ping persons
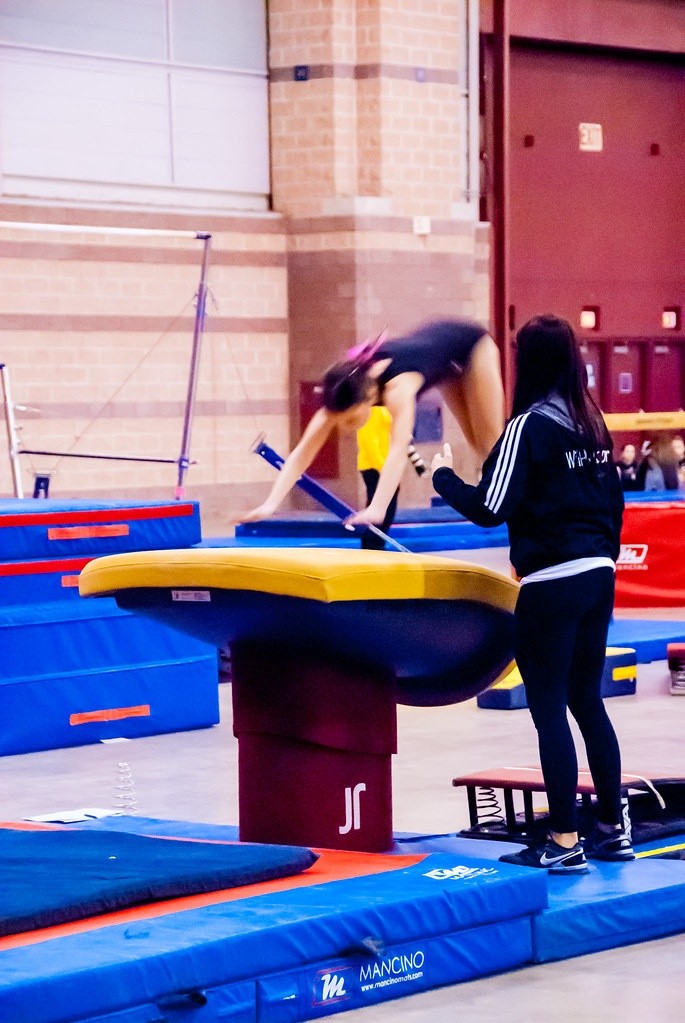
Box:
[238,317,504,524]
[615,443,639,491]
[430,312,632,873]
[636,431,685,490]
[357,404,427,551]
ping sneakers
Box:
[499,834,589,875]
[580,825,635,861]
